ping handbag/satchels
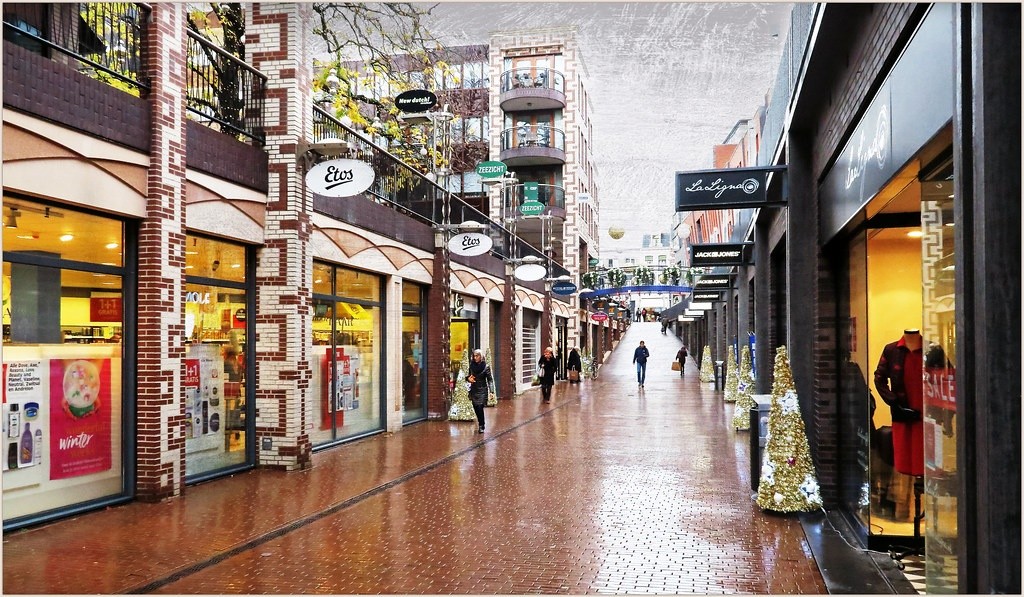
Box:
[569,367,578,380]
[672,362,681,371]
[661,328,665,332]
[539,367,544,377]
[678,350,681,358]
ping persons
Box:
[873,328,956,473]
[567,345,582,383]
[634,306,661,322]
[633,341,650,388]
[537,346,558,404]
[661,314,669,335]
[465,348,495,432]
[675,346,688,376]
[224,348,245,446]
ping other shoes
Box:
[638,384,642,386]
[546,401,550,404]
[642,385,644,387]
[543,400,546,403]
[479,426,484,433]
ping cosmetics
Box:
[7,401,43,470]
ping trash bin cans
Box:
[714,361,724,394]
[748,394,772,501]
